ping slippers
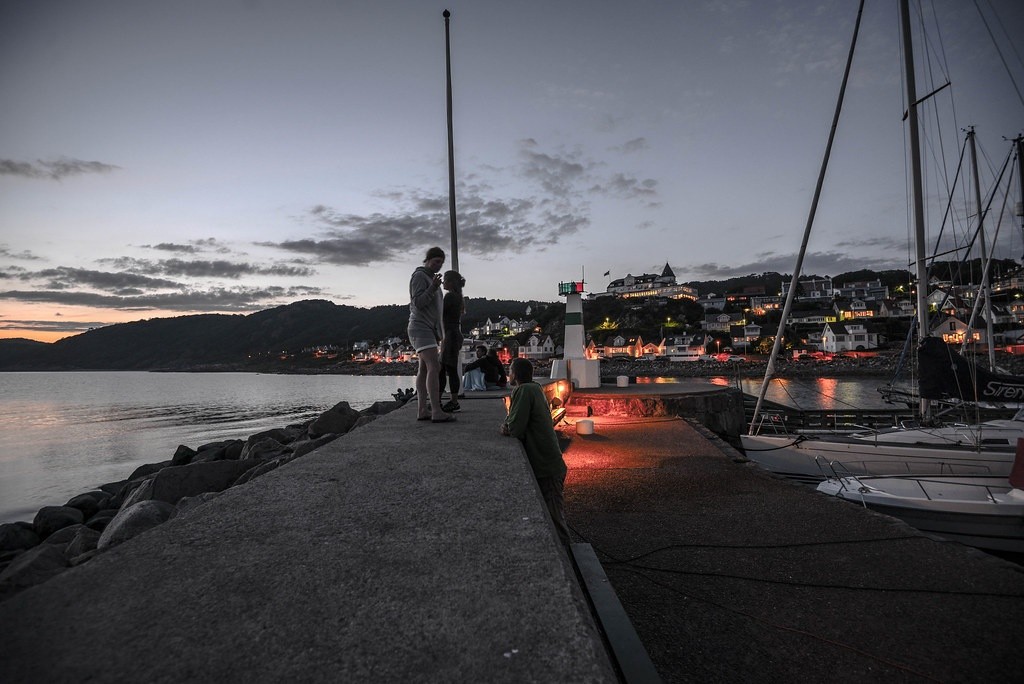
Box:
[417,413,432,420]
[431,411,457,423]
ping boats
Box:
[814,456,1024,517]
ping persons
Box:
[500,358,574,544]
[391,388,414,404]
[462,345,507,392]
[407,247,457,423]
[427,270,466,413]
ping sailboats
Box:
[739,0,1024,485]
[875,125,1024,416]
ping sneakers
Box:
[442,399,461,413]
[428,399,442,411]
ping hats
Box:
[423,247,444,263]
